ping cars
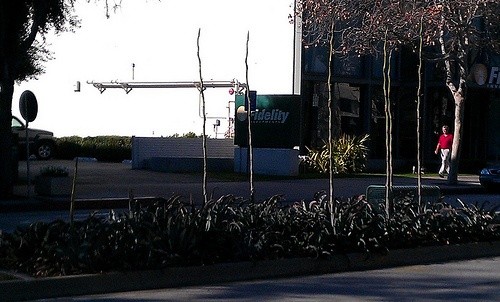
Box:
[479,160,500,190]
[10,126,58,159]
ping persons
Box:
[434,125,454,177]
